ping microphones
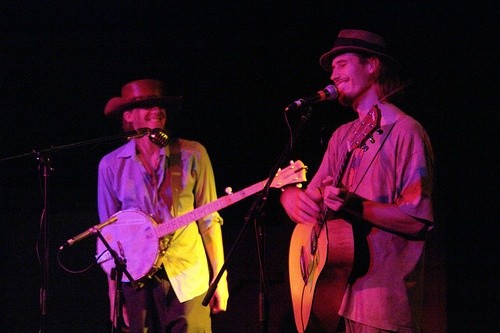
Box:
[139,128,170,147]
[285,85,338,110]
[57,217,117,252]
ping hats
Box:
[105,79,181,117]
[319,30,401,75]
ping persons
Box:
[281,30,435,333]
[98,81,230,333]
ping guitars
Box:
[96,160,308,286]
[288,104,384,333]
